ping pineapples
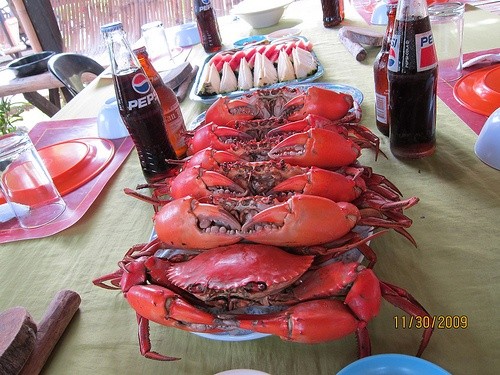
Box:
[198,47,317,96]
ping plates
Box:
[232,27,302,48]
[189,35,324,104]
[187,82,364,132]
[149,223,375,343]
[336,353,453,375]
[452,63,500,117]
[0,136,116,210]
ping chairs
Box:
[47,53,103,98]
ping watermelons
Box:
[209,39,313,74]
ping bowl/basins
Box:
[8,50,56,78]
[175,22,200,47]
[473,106,500,170]
[370,3,391,25]
[229,0,287,29]
[97,96,130,140]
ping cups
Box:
[140,21,176,76]
[428,2,466,84]
[0,131,67,229]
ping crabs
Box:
[91,85,437,357]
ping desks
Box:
[0,0,500,375]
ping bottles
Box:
[100,21,179,185]
[321,0,345,28]
[193,0,223,54]
[373,0,399,137]
[386,0,438,158]
[131,44,192,158]
